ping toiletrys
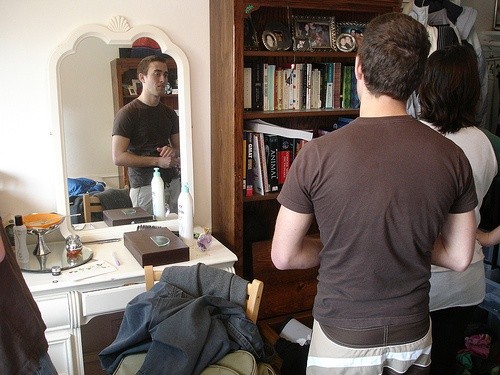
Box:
[3,217,14,252]
[13,215,30,263]
[151,168,164,220]
[178,183,194,246]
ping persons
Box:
[271,11,480,375]
[417,47,500,375]
[0,215,58,375]
[300,24,322,47]
[112,56,180,214]
[339,28,363,50]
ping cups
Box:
[166,203,170,216]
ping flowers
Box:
[194,228,213,252]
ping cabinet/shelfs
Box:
[110,58,178,188]
[209,0,403,375]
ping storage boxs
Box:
[123,227,190,268]
[102,207,154,227]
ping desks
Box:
[19,224,238,375]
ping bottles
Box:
[177,184,194,247]
[13,215,30,264]
[151,168,165,220]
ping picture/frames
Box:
[243,5,371,52]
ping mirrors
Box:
[51,15,196,237]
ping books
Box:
[242,117,355,196]
[244,62,360,113]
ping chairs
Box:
[83,185,130,222]
[144,265,264,326]
